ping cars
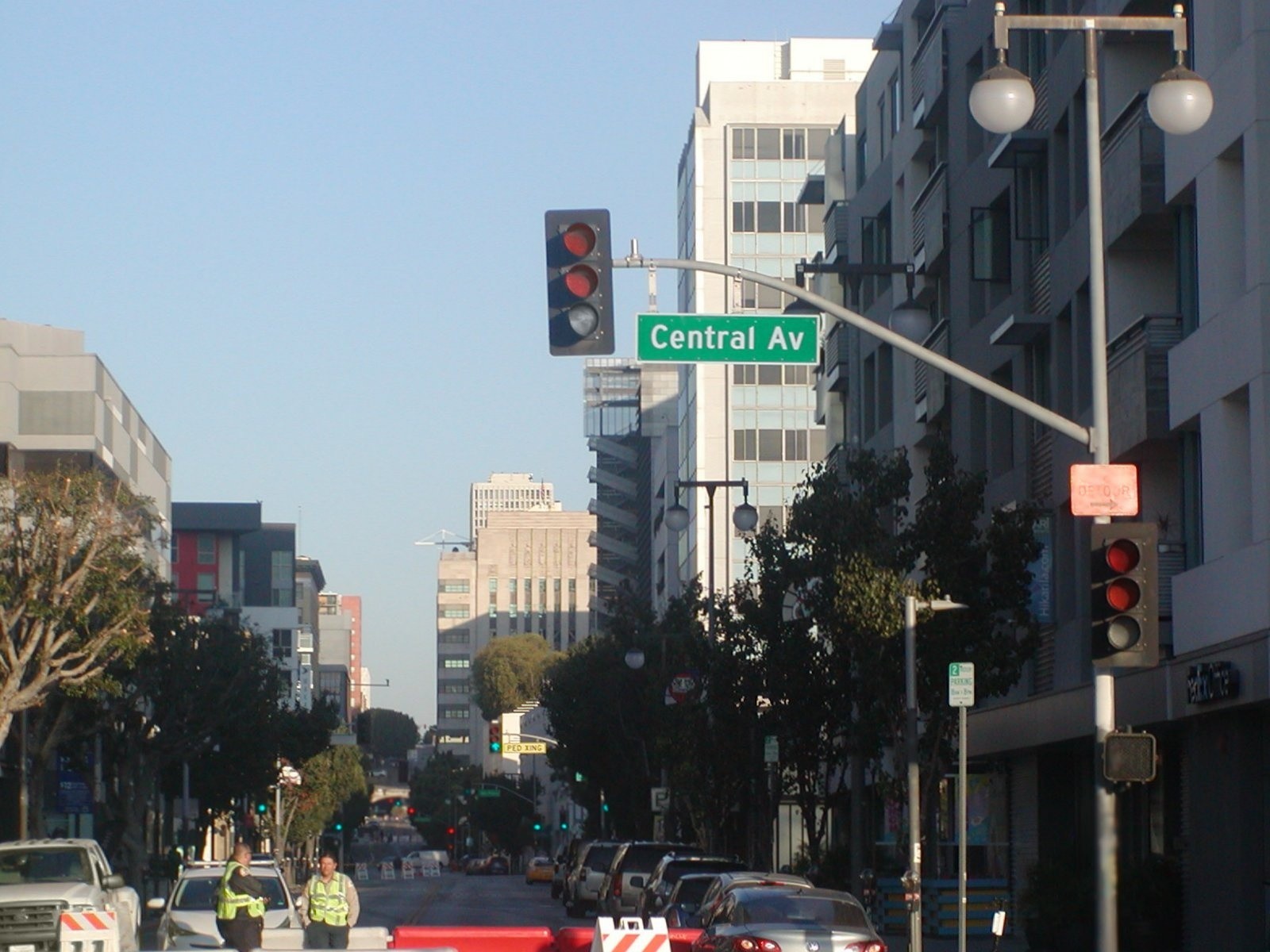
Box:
[527,857,556,885]
[158,859,306,950]
[379,851,512,876]
[698,872,838,928]
[691,886,887,952]
[654,873,720,934]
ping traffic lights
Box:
[389,797,402,807]
[333,821,343,833]
[406,807,418,815]
[543,208,618,357]
[489,723,502,755]
[1102,732,1158,785]
[532,814,543,832]
[255,796,269,816]
[447,826,455,836]
[559,809,569,831]
[463,780,472,797]
[1091,518,1160,671]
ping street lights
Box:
[663,475,760,844]
[906,593,965,952]
[966,1,1215,952]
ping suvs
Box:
[565,841,626,917]
[594,841,708,920]
[630,852,751,928]
[549,842,574,898]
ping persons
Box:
[301,851,360,950]
[551,855,568,900]
[168,843,192,888]
[215,842,271,952]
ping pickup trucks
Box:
[0,836,142,952]
